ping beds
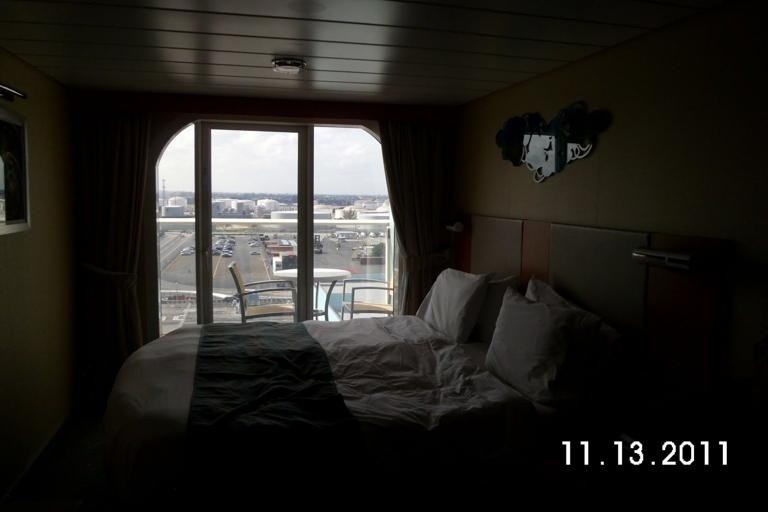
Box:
[102,315,642,508]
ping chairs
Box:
[228,260,395,325]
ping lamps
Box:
[445,222,465,234]
[270,57,307,75]
[632,247,694,284]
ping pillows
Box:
[413,265,623,411]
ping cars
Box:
[180,245,195,256]
[248,232,270,247]
[273,233,277,239]
[250,251,261,255]
[212,235,235,258]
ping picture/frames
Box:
[0,104,31,237]
